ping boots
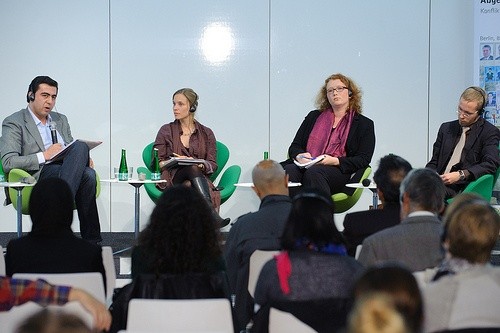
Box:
[191,177,231,230]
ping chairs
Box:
[137,140,240,205]
[331,164,373,212]
[0,155,101,215]
[446,168,500,204]
[0,247,327,333]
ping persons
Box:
[0,154,500,333]
[282,73,375,210]
[1,76,103,251]
[152,88,231,229]
[424,85,500,201]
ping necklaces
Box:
[181,127,195,136]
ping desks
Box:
[100,179,167,255]
[346,183,378,210]
[0,180,35,237]
[233,182,301,188]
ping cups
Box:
[127,166,133,179]
[114,168,119,179]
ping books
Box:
[159,158,205,170]
[50,138,103,162]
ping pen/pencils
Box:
[296,156,316,160]
[173,153,188,158]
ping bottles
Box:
[264,152,268,160]
[0,152,6,182]
[150,150,161,181]
[119,149,128,181]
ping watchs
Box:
[458,170,465,180]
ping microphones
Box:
[362,179,371,187]
[49,121,57,144]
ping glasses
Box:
[327,86,349,94]
[456,106,478,118]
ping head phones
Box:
[27,84,35,100]
[347,80,353,97]
[470,87,485,115]
[189,94,197,112]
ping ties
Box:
[444,126,471,173]
